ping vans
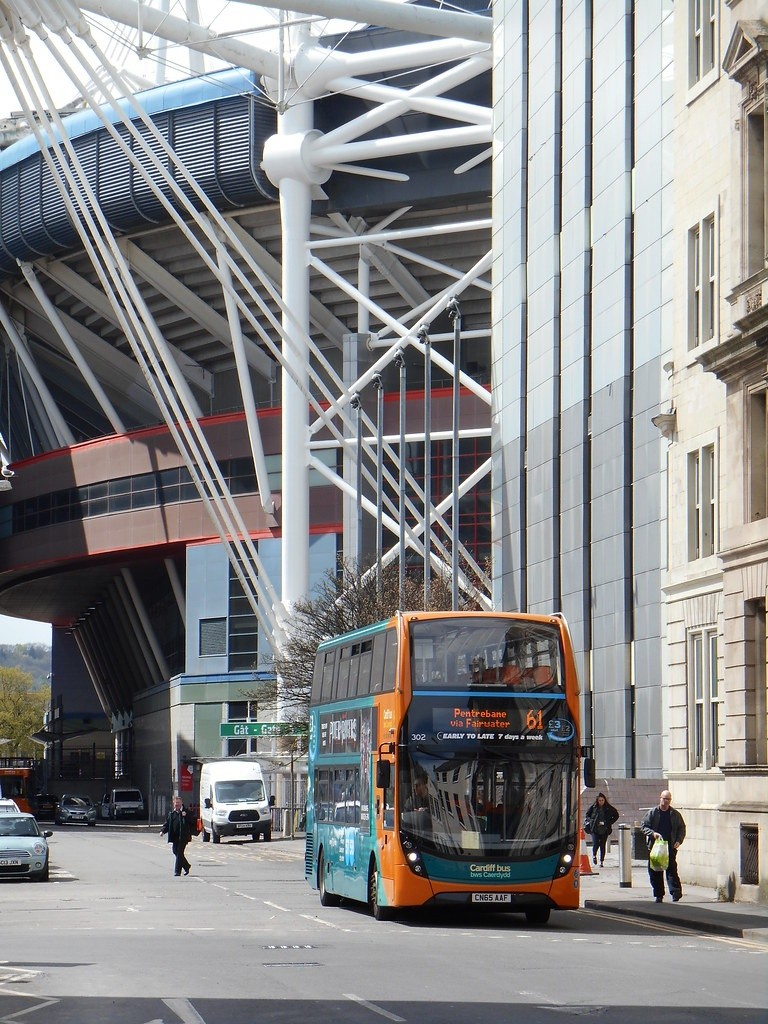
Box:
[110,788,144,820]
[200,760,276,844]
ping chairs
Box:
[533,665,553,685]
[504,665,521,684]
[490,667,503,682]
[520,667,534,680]
[482,669,490,684]
[456,670,481,684]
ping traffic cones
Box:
[579,828,600,876]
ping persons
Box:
[159,797,197,876]
[402,774,440,813]
[641,791,686,903]
[583,793,619,867]
[10,781,22,796]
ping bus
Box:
[0,768,36,814]
[304,609,596,925]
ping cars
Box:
[54,793,96,827]
[101,793,113,819]
[0,812,53,882]
[0,798,25,829]
[32,792,60,820]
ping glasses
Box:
[413,784,420,790]
[662,797,672,800]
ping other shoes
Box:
[599,864,603,867]
[672,894,682,902]
[174,872,180,876]
[654,896,662,903]
[593,856,597,865]
[184,865,192,875]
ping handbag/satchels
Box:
[583,820,591,834]
[650,835,669,871]
[190,819,202,836]
